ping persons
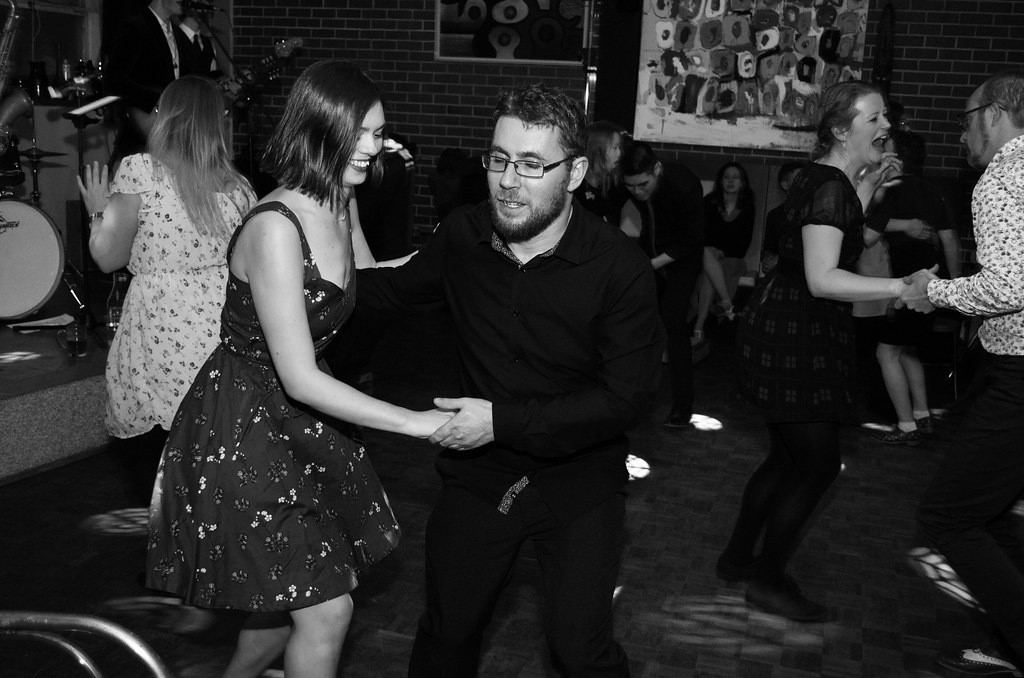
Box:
[143,60,459,678]
[852,131,980,447]
[574,121,806,478]
[718,83,939,627]
[405,81,657,678]
[77,74,259,438]
[896,76,1024,678]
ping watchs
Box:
[87,211,103,229]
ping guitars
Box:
[131,37,303,138]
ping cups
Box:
[57,323,87,357]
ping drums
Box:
[0,125,26,195]
[0,188,66,322]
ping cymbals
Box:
[19,147,69,160]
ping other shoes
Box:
[689,337,708,351]
[714,552,754,582]
[748,575,829,622]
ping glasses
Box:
[481,148,575,176]
[954,101,1006,130]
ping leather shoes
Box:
[871,427,920,448]
[663,404,693,428]
[912,415,935,434]
[936,647,1020,676]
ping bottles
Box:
[62,59,71,82]
[73,58,101,102]
[106,272,130,356]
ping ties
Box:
[192,34,202,53]
[163,25,178,69]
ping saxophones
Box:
[0,0,34,159]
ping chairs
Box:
[737,166,769,287]
